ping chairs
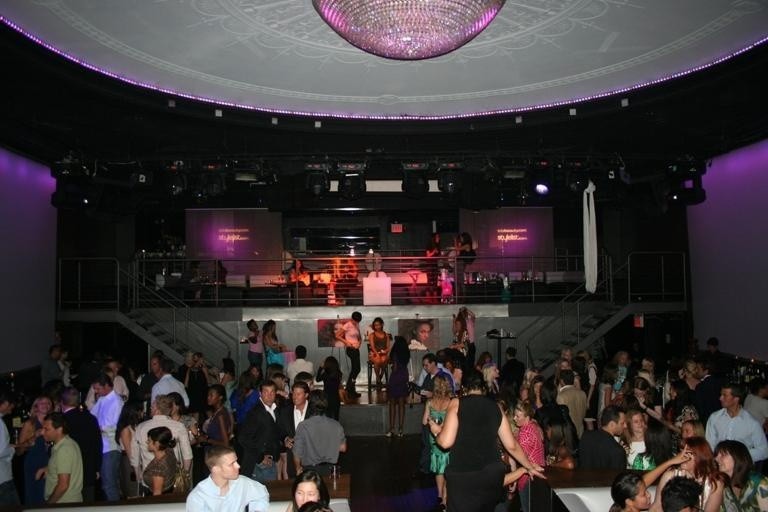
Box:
[367,344,388,391]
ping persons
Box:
[422,372,459,512]
[289,259,312,305]
[409,351,462,480]
[181,262,201,304]
[474,334,766,509]
[335,258,359,306]
[449,231,472,302]
[329,306,474,404]
[425,232,442,304]
[386,335,409,437]
[215,259,227,305]
[623,243,643,302]
[239,319,282,367]
[427,369,547,511]
[1,347,344,511]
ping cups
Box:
[327,464,342,480]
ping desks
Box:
[529,467,672,512]
[488,333,517,369]
[268,282,357,305]
[262,474,351,502]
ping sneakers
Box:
[352,391,361,397]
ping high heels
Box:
[385,431,394,438]
[397,430,403,437]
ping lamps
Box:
[311,1,506,60]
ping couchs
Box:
[313,275,356,305]
[247,275,289,306]
[357,272,428,304]
[171,272,247,304]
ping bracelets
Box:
[434,432,441,438]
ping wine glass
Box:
[431,415,442,442]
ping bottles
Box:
[78,391,84,412]
[11,427,20,446]
